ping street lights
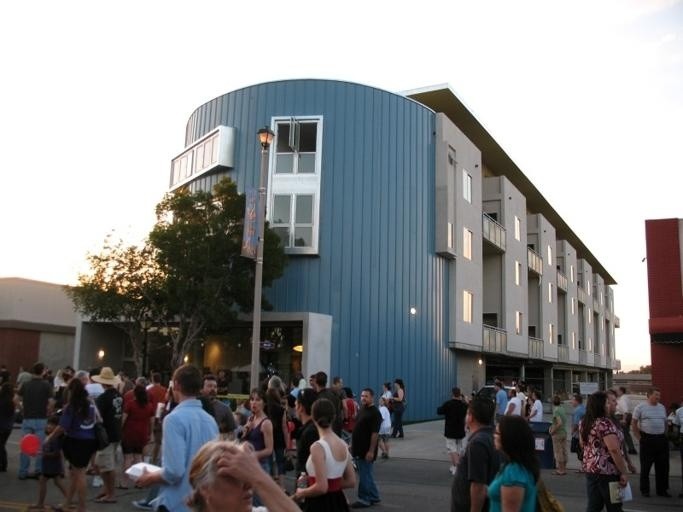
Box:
[249,127,275,395]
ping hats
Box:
[92,367,121,386]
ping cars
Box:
[470,385,529,418]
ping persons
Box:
[437,374,683,512]
[1,360,408,511]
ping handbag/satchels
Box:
[536,479,564,512]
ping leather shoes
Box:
[643,491,651,498]
[656,490,671,498]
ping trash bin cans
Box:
[527,421,556,469]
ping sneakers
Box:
[382,452,389,458]
[450,465,457,475]
[350,498,381,509]
[551,469,567,475]
[28,475,129,512]
[628,463,636,472]
[132,499,154,510]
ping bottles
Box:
[297,472,307,503]
